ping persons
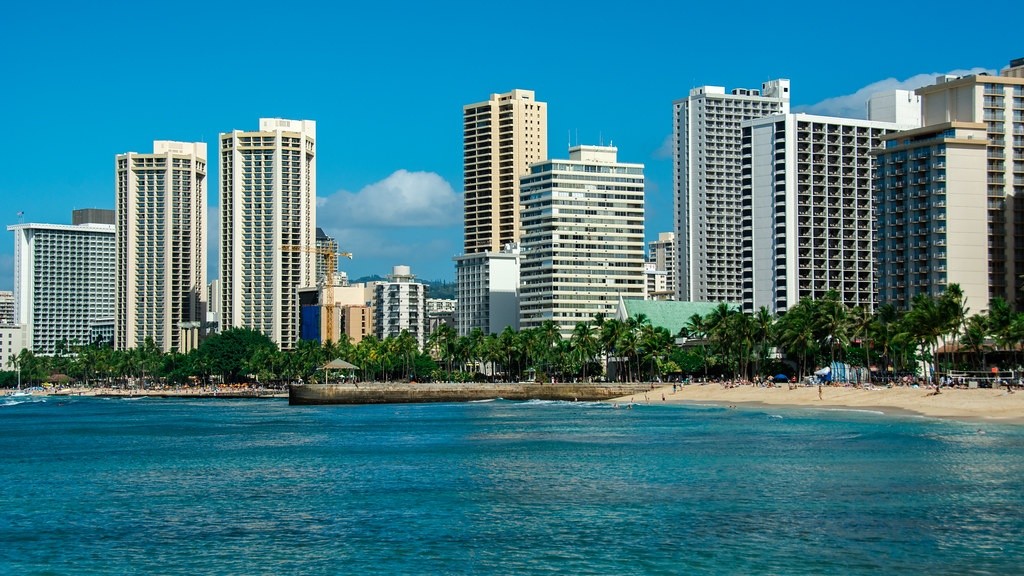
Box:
[792,376,797,383]
[753,374,774,384]
[652,381,683,392]
[353,377,359,384]
[686,375,689,384]
[614,393,666,408]
[552,377,558,383]
[5,386,55,397]
[58,384,63,389]
[214,382,254,392]
[157,379,204,389]
[66,383,70,388]
[700,377,752,390]
[872,373,1024,387]
[338,378,348,384]
[578,376,601,382]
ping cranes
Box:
[280,240,352,344]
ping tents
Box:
[316,358,360,384]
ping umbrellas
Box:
[775,374,787,378]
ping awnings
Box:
[935,341,1024,353]
